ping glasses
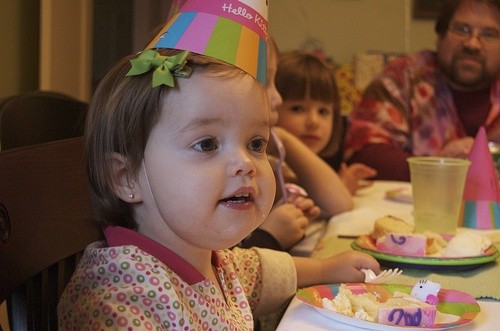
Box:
[444,22,500,47]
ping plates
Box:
[294,282,480,331]
[385,186,412,203]
[352,239,499,267]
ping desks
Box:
[275,179,500,331]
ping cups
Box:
[408,156,470,234]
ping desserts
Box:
[371,216,427,256]
[323,284,436,328]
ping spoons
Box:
[271,127,289,202]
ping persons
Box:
[274,51,376,221]
[341,0,500,182]
[227,32,321,253]
[57,48,384,331]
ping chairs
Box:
[0,90,103,331]
